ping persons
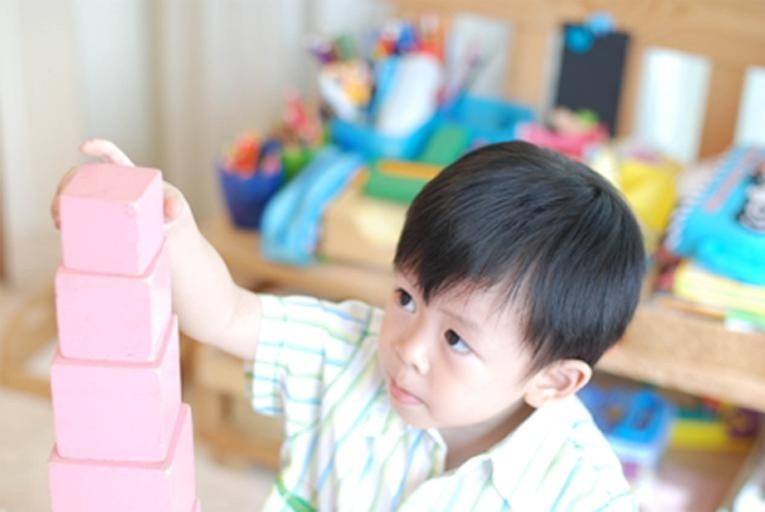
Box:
[52,140,644,512]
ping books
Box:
[665,141,765,335]
